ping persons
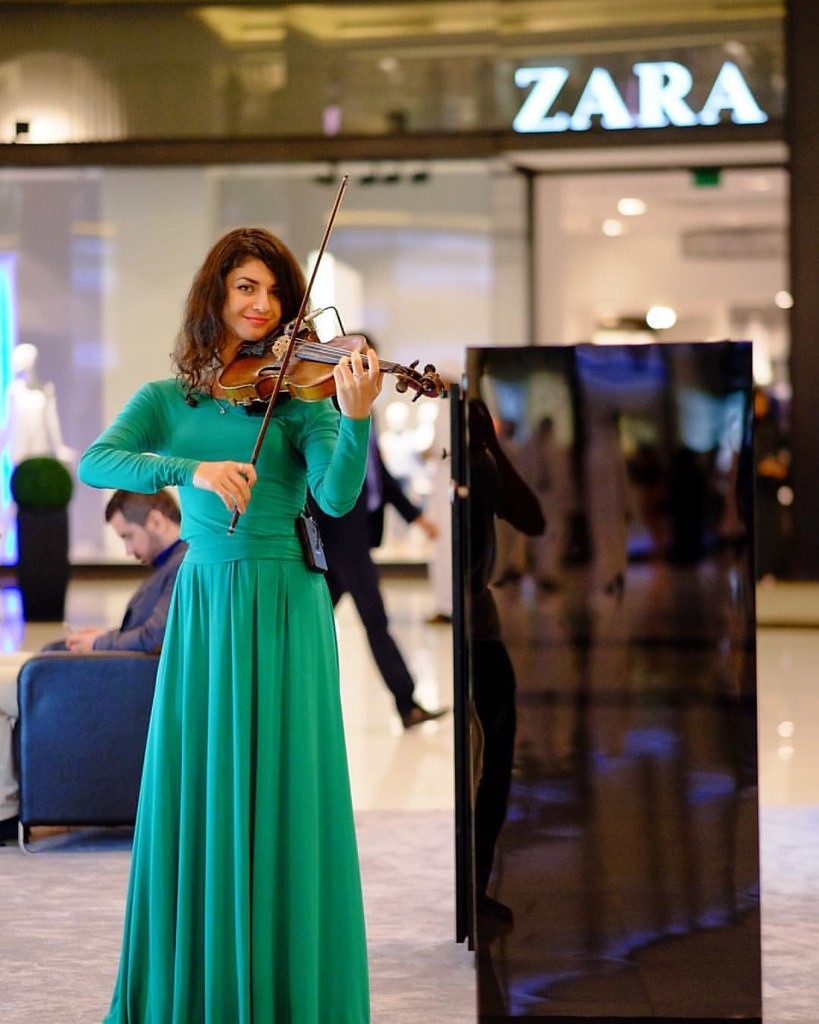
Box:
[451,386,551,945]
[0,486,190,846]
[305,333,449,732]
[77,229,385,1024]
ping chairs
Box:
[13,640,161,853]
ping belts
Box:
[422,448,451,459]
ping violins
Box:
[216,322,447,405]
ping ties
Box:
[368,426,382,507]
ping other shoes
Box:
[426,615,453,625]
[403,702,446,728]
[0,815,32,845]
[462,895,514,943]
